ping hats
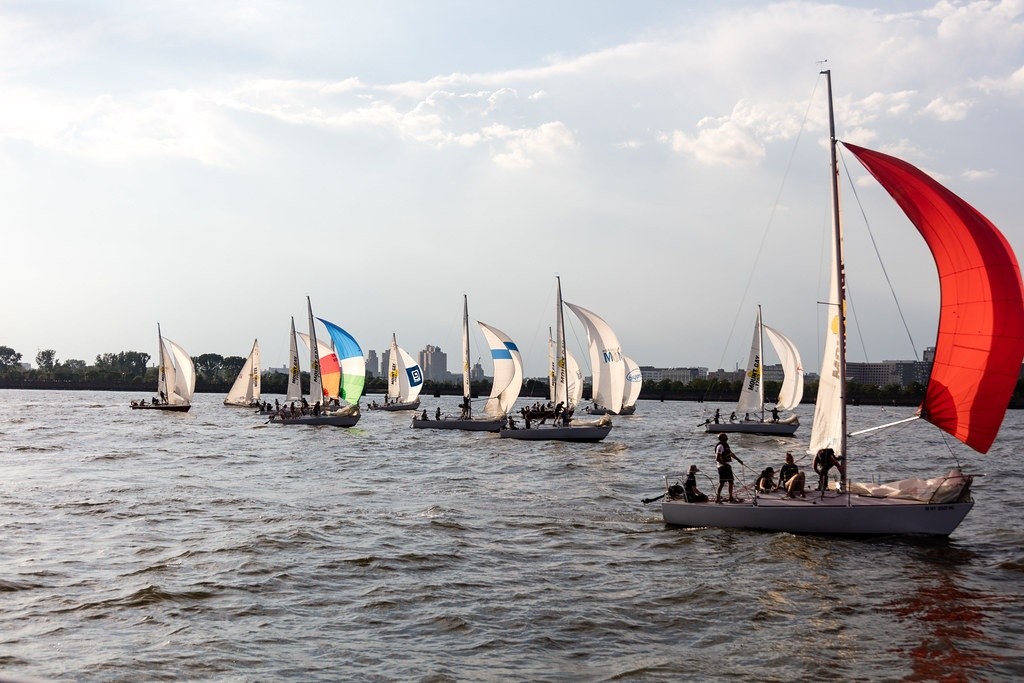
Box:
[690,465,700,472]
[718,433,728,440]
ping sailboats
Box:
[369,334,424,411]
[662,69,1024,537]
[500,276,614,440]
[563,301,643,415]
[705,305,804,435]
[224,317,365,411]
[270,295,366,426]
[411,294,522,432]
[520,326,584,420]
[131,322,196,412]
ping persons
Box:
[372,400,378,407]
[140,399,150,406]
[256,400,259,406]
[384,393,390,404]
[267,403,272,411]
[435,407,444,421]
[682,434,744,504]
[508,416,520,429]
[593,402,598,409]
[259,405,265,412]
[300,398,310,416]
[280,404,288,419]
[262,400,266,406]
[310,401,322,416]
[275,399,281,412]
[152,396,159,404]
[391,400,394,404]
[421,409,428,420]
[290,402,295,418]
[463,394,471,418]
[516,401,565,429]
[714,407,783,424]
[160,390,167,404]
[755,448,842,498]
